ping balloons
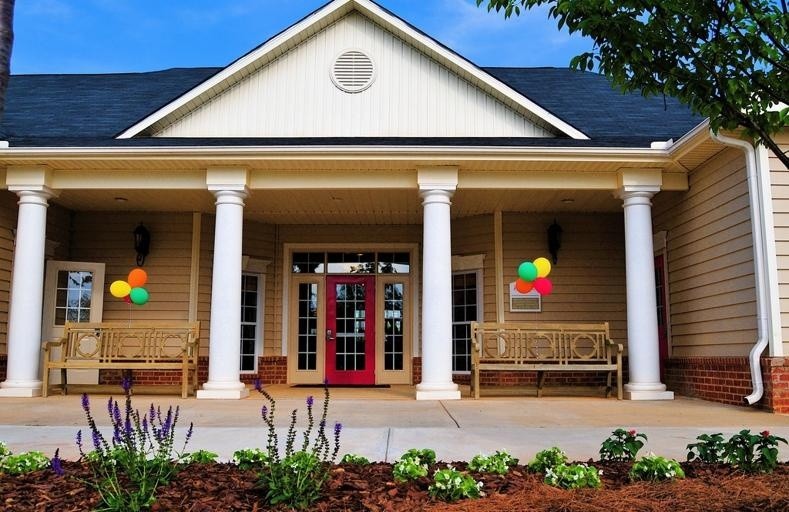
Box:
[531,276,554,296]
[123,294,134,305]
[515,279,533,295]
[129,286,149,304]
[128,268,148,289]
[517,261,537,282]
[109,280,131,297]
[532,257,552,278]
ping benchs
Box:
[41,319,202,399]
[469,320,624,400]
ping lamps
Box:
[132,220,152,267]
[546,219,564,265]
